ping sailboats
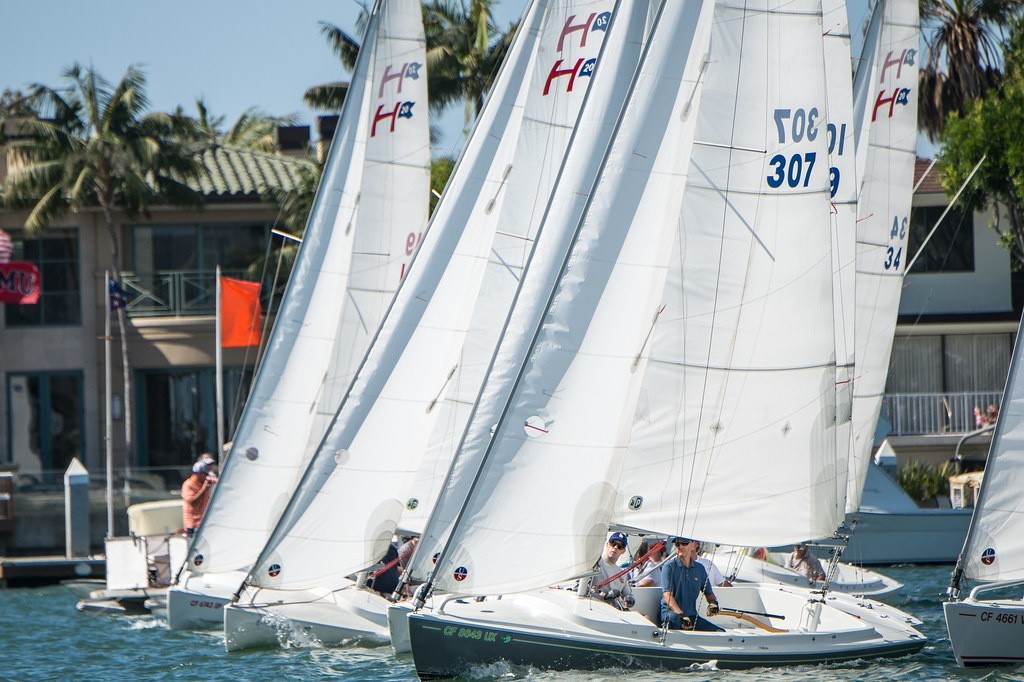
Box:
[74,0,1024,670]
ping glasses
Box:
[675,541,690,548]
[608,541,624,551]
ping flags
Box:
[215,276,262,349]
[105,277,129,312]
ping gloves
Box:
[605,589,620,600]
[707,601,719,616]
[679,613,693,630]
[623,597,635,607]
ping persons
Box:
[354,535,421,603]
[785,541,825,579]
[589,532,733,632]
[973,405,998,436]
[182,453,219,538]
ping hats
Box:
[192,462,208,473]
[202,458,215,465]
[671,536,698,543]
[608,533,628,545]
[647,542,666,550]
